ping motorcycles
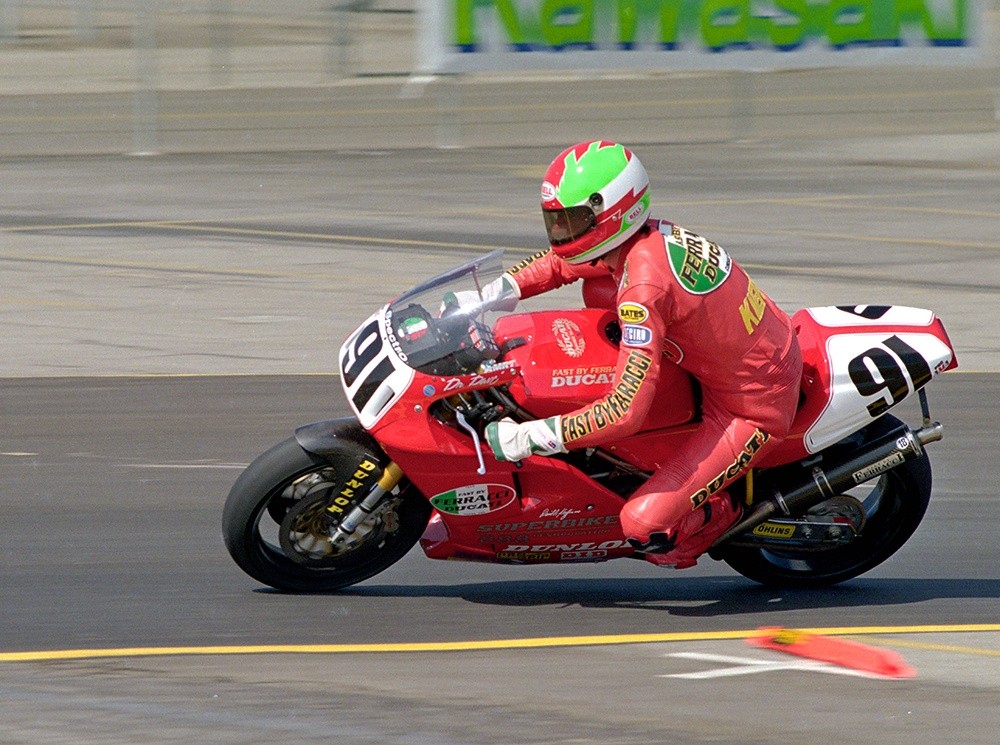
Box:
[220,250,968,594]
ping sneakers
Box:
[644,490,745,569]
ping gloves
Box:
[435,273,522,325]
[484,414,570,462]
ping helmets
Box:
[539,139,653,265]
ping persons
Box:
[437,140,804,571]
